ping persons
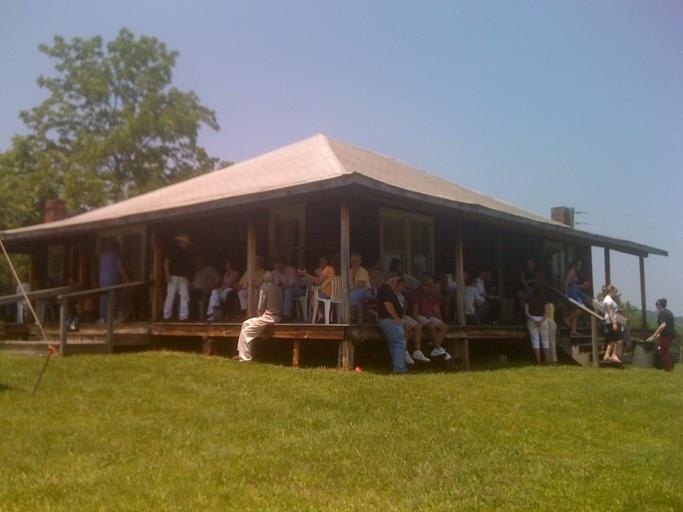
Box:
[383,258,410,312]
[15,271,32,327]
[519,257,541,295]
[440,263,594,328]
[645,299,676,372]
[97,239,131,325]
[35,275,63,328]
[542,291,558,363]
[392,273,431,365]
[232,269,284,362]
[377,270,410,376]
[563,258,590,338]
[521,282,551,365]
[409,274,453,362]
[600,283,625,364]
[187,252,381,325]
[160,232,195,322]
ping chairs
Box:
[292,277,312,320]
[312,276,342,325]
[366,279,378,322]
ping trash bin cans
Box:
[631,340,657,368]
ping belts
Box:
[266,313,281,316]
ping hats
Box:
[174,234,193,247]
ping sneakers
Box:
[404,350,414,365]
[603,354,622,364]
[412,349,430,362]
[430,345,451,360]
[393,368,417,374]
